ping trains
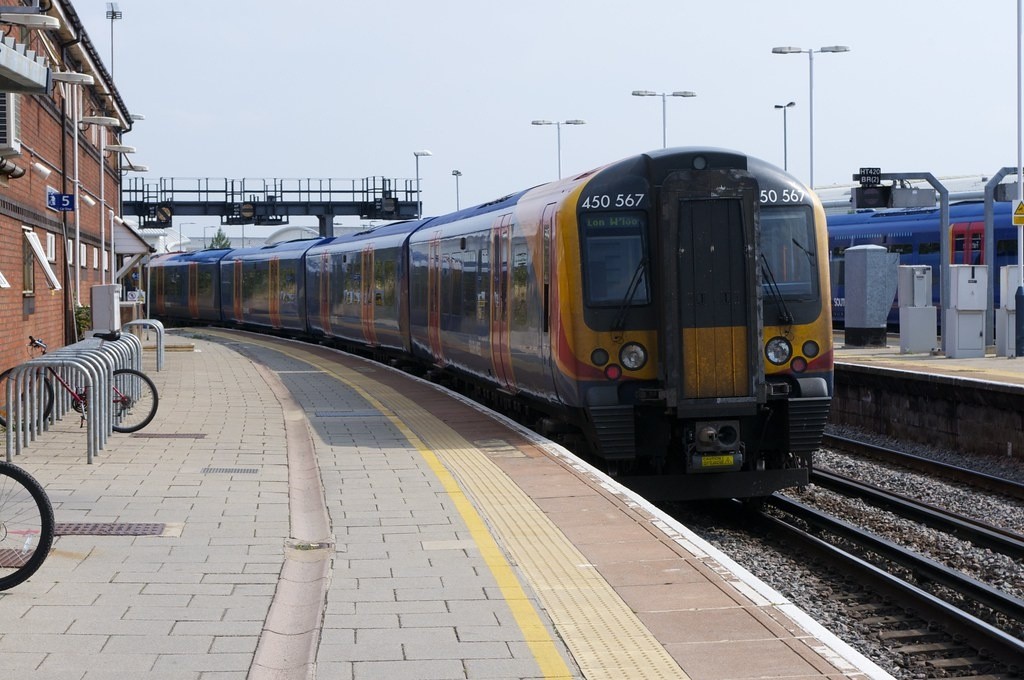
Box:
[123,147,836,500]
[826,201,1018,330]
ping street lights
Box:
[770,45,853,192]
[452,169,463,212]
[774,101,796,173]
[631,89,696,148]
[530,119,587,182]
[413,149,433,220]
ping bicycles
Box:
[0,459,55,592]
[0,329,159,434]
